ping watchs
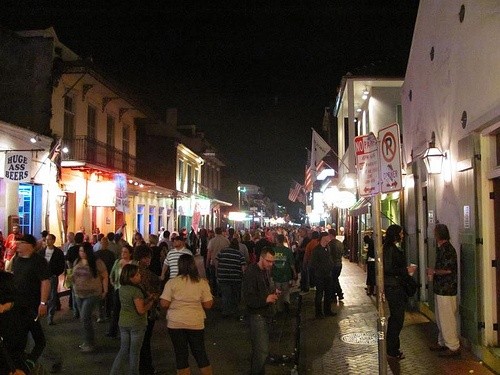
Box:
[40,301,47,305]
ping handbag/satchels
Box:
[404,279,416,297]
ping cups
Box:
[408,263,417,276]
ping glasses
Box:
[263,257,274,263]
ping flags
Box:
[304,150,325,193]
[240,184,257,195]
[288,180,302,202]
[48,139,62,184]
[310,131,331,182]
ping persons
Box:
[214,239,248,320]
[427,223,462,358]
[240,246,283,375]
[159,253,214,375]
[3,224,25,271]
[383,224,418,359]
[363,226,377,296]
[110,264,157,375]
[38,234,65,325]
[131,245,162,375]
[8,234,52,360]
[0,271,47,375]
[36,230,50,250]
[64,242,108,352]
[64,223,344,319]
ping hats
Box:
[171,236,183,241]
[317,232,329,242]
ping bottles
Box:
[428,265,433,283]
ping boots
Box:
[176,366,190,375]
[200,365,212,375]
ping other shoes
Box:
[97,317,103,323]
[316,313,325,319]
[107,332,118,338]
[77,343,97,353]
[429,344,446,351]
[438,349,460,357]
[387,350,403,359]
[325,311,337,317]
